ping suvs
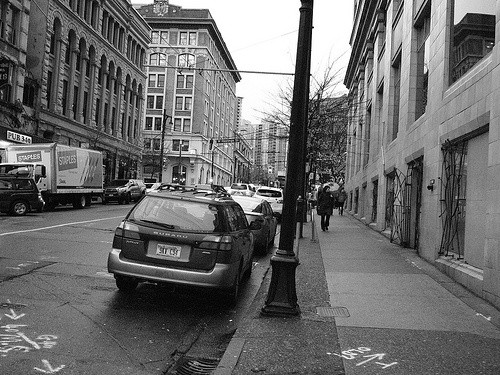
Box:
[252,185,284,222]
[101,178,146,205]
[144,183,161,195]
[107,182,256,309]
[224,183,261,197]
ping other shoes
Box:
[323,228,325,231]
[325,226,328,230]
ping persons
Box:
[337,187,347,216]
[316,186,333,232]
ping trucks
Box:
[0,142,106,209]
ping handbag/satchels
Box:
[329,194,334,206]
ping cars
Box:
[228,195,278,258]
[0,173,46,217]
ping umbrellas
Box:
[323,182,340,191]
